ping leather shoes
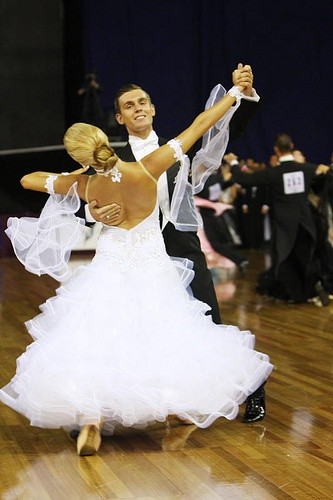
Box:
[244,388,265,422]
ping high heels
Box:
[77,415,104,456]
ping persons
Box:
[77,70,106,129]
[75,64,266,424]
[192,137,333,306]
[0,62,274,456]
